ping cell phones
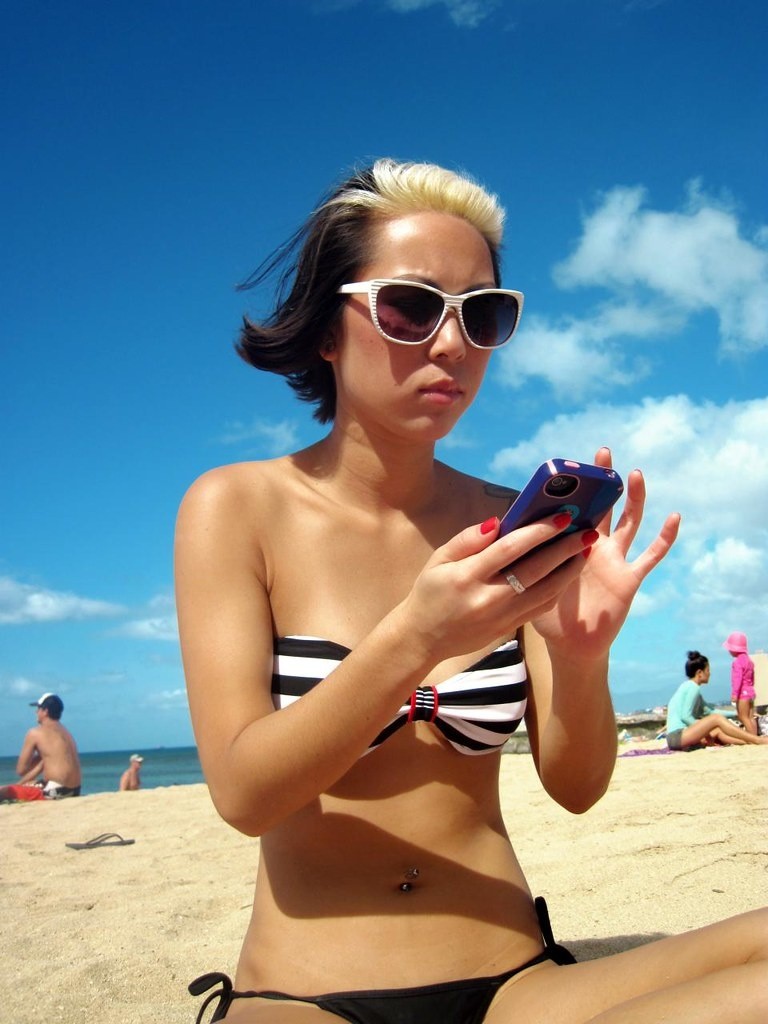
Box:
[490,458,624,574]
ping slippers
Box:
[65,833,135,849]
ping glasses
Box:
[334,278,527,351]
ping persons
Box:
[0,692,81,800]
[723,631,758,735]
[174,162,768,1024]
[666,651,768,751]
[120,753,143,790]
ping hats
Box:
[721,633,750,654]
[129,755,145,763]
[30,692,63,712]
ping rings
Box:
[506,573,526,594]
